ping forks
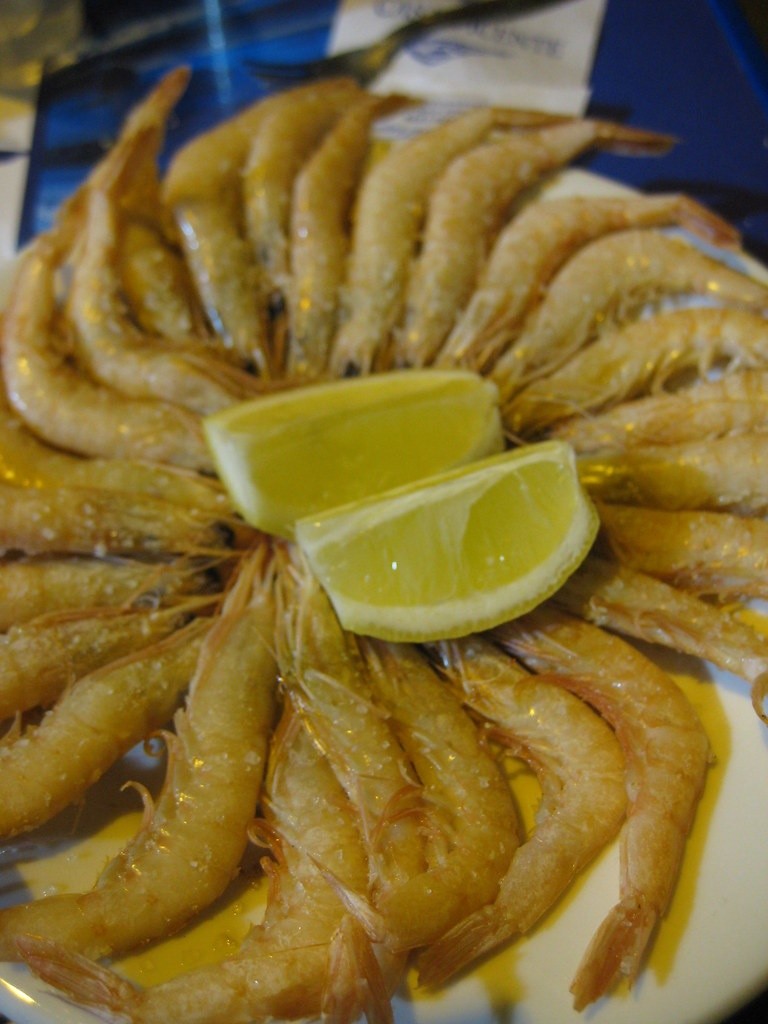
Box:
[246,0,570,86]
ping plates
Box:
[0,121,768,1024]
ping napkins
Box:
[325,0,607,116]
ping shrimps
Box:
[1,63,768,1024]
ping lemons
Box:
[294,438,600,642]
[203,366,507,537]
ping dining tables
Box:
[2,0,768,1022]
